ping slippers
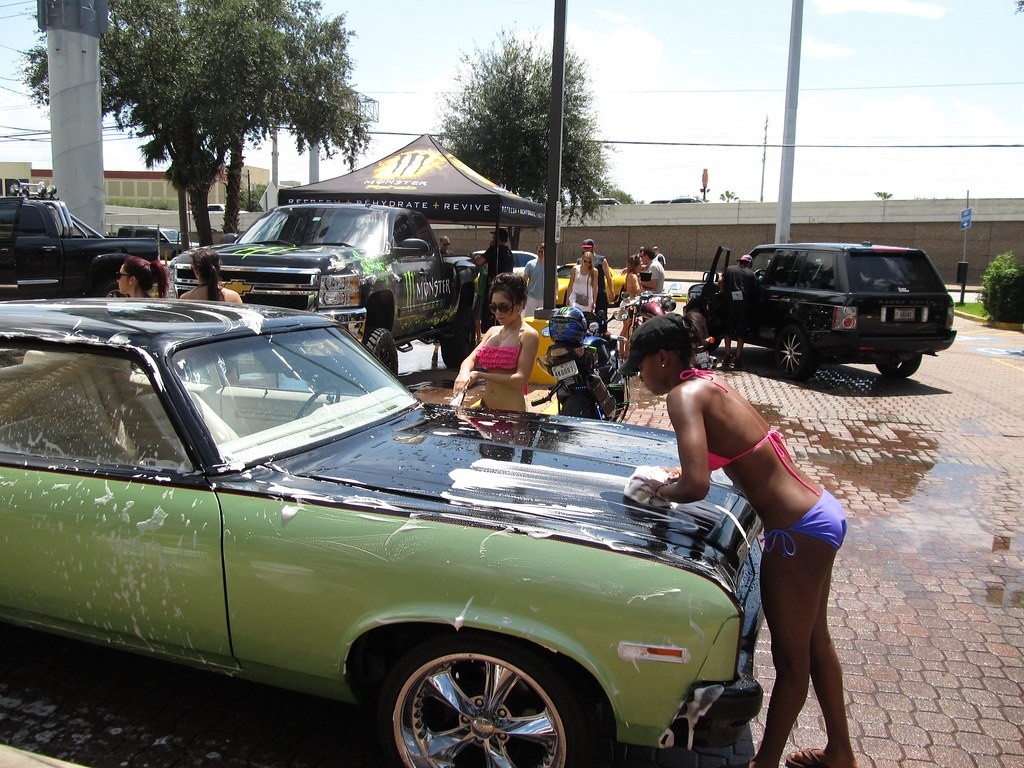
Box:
[786,748,826,768]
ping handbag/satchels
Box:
[576,294,588,306]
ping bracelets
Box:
[656,484,669,499]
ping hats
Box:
[490,228,508,238]
[617,314,689,374]
[582,239,594,248]
[736,254,754,267]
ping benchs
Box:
[0,357,121,444]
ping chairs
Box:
[0,410,132,462]
[117,389,240,463]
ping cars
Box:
[469,249,563,278]
[591,198,622,205]
[557,262,628,311]
[0,300,766,768]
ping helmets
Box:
[549,307,587,343]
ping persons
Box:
[456,405,531,462]
[523,243,558,317]
[477,229,514,334]
[180,249,242,302]
[454,273,539,412]
[718,254,758,368]
[619,314,859,768]
[117,257,168,298]
[620,247,666,358]
[566,239,615,336]
[432,236,454,361]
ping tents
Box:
[277,134,546,325]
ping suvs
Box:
[683,238,958,383]
[650,200,672,205]
[116,225,199,260]
[668,197,704,203]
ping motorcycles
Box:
[607,284,717,374]
[531,306,631,424]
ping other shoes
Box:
[723,354,732,367]
[734,360,741,370]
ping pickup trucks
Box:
[0,178,161,304]
[186,204,250,214]
[167,203,478,383]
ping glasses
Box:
[115,272,134,280]
[489,302,516,313]
[584,260,591,264]
[443,242,450,245]
[538,250,543,254]
